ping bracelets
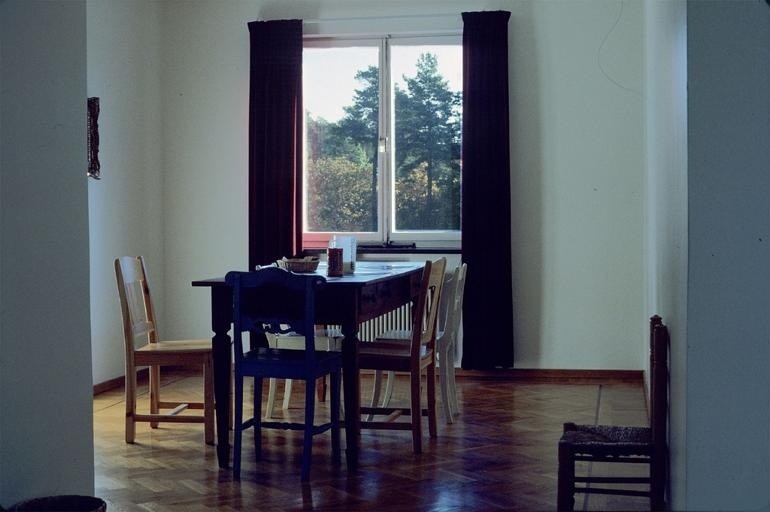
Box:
[286,260,319,273]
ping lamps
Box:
[556,313,672,511]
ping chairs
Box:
[116,257,233,445]
[190,258,468,479]
[556,313,672,511]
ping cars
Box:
[328,235,356,274]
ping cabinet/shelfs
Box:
[327,248,344,277]
